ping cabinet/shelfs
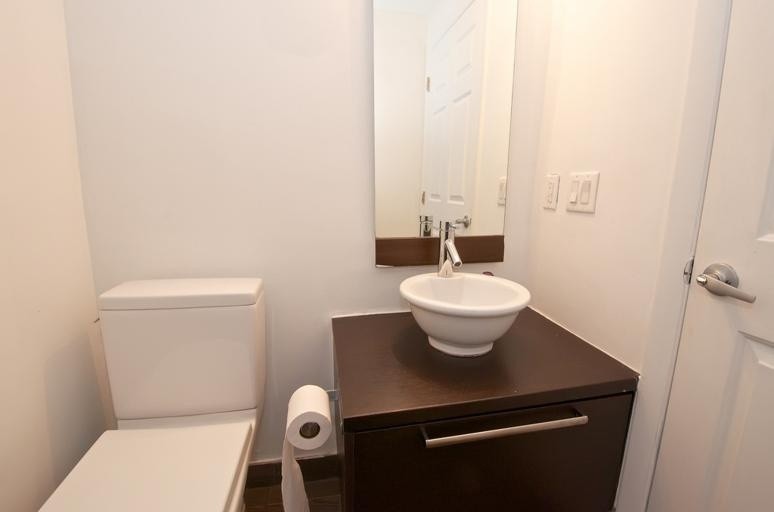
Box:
[351,395,638,511]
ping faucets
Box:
[439,219,463,277]
[419,214,435,238]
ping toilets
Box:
[36,274,268,512]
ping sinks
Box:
[400,270,531,358]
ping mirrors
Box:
[371,1,519,265]
